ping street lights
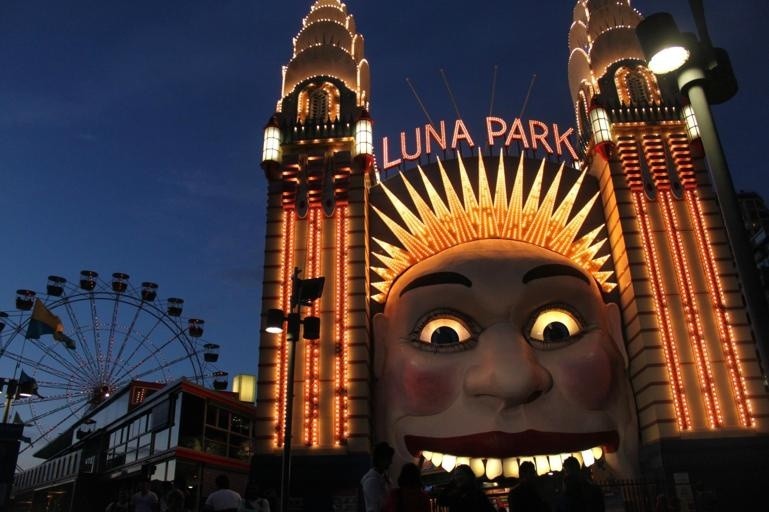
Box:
[0,367,38,423]
[265,268,325,511]
[634,12,768,380]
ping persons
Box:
[349,442,432,509]
[105,478,191,512]
[440,465,496,512]
[200,474,241,512]
[507,456,605,512]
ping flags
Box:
[25,298,63,340]
[16,368,48,402]
